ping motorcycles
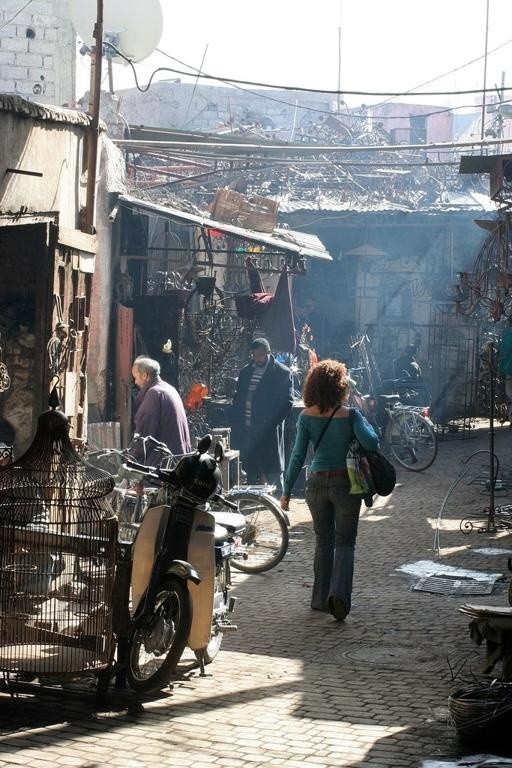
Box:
[76,451,211,585]
[124,433,238,695]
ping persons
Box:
[229,338,293,506]
[120,357,191,468]
[394,344,423,380]
[277,358,382,620]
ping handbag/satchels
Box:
[344,438,376,508]
[366,447,397,497]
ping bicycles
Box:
[87,433,290,573]
[346,366,438,472]
